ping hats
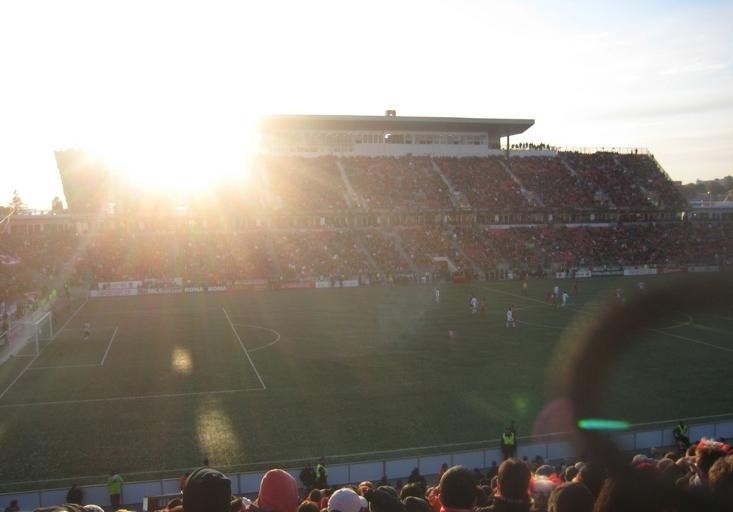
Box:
[328,487,369,512]
